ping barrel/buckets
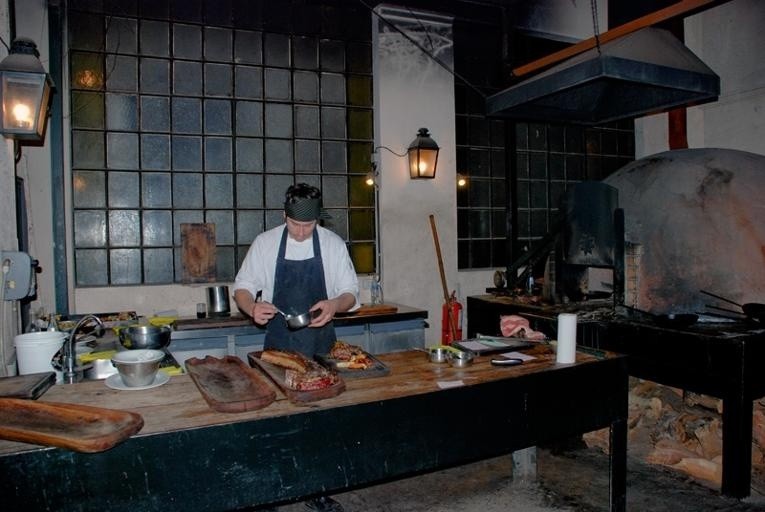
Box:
[12,330,69,385]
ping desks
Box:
[465,289,765,499]
[1,330,631,512]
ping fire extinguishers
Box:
[441,290,463,346]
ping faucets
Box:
[64,314,103,383]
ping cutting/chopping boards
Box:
[353,304,399,314]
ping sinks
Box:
[81,358,163,381]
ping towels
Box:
[500,313,546,341]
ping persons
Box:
[230,183,361,512]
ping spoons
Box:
[276,308,293,321]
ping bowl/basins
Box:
[284,312,312,331]
[120,325,172,350]
[447,352,474,368]
[431,348,451,363]
[112,348,165,385]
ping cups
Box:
[196,302,206,320]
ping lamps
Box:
[0,37,57,146]
[457,173,471,190]
[365,172,380,188]
[408,128,440,180]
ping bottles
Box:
[47,313,63,354]
[26,308,41,333]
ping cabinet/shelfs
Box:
[332,314,370,357]
[173,316,233,360]
[233,312,267,363]
[370,301,428,356]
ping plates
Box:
[1,397,144,453]
[185,355,277,413]
[450,338,536,356]
[314,347,390,380]
[104,373,171,392]
[246,350,346,403]
